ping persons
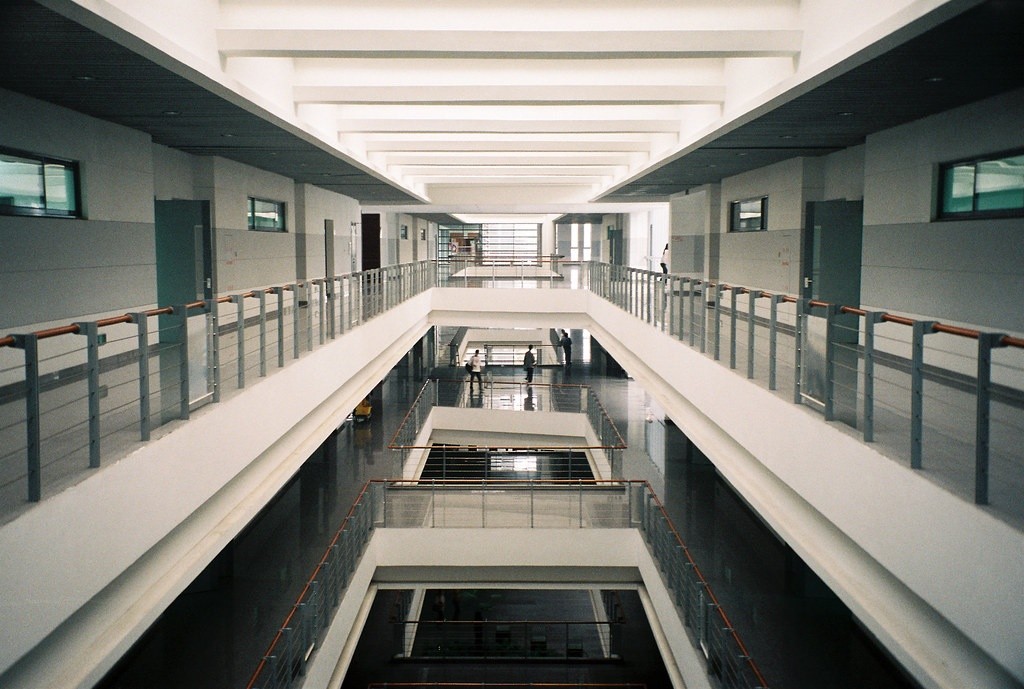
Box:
[468,350,486,390]
[560,329,573,365]
[524,345,535,382]
[660,244,668,282]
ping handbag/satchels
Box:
[465,363,472,376]
